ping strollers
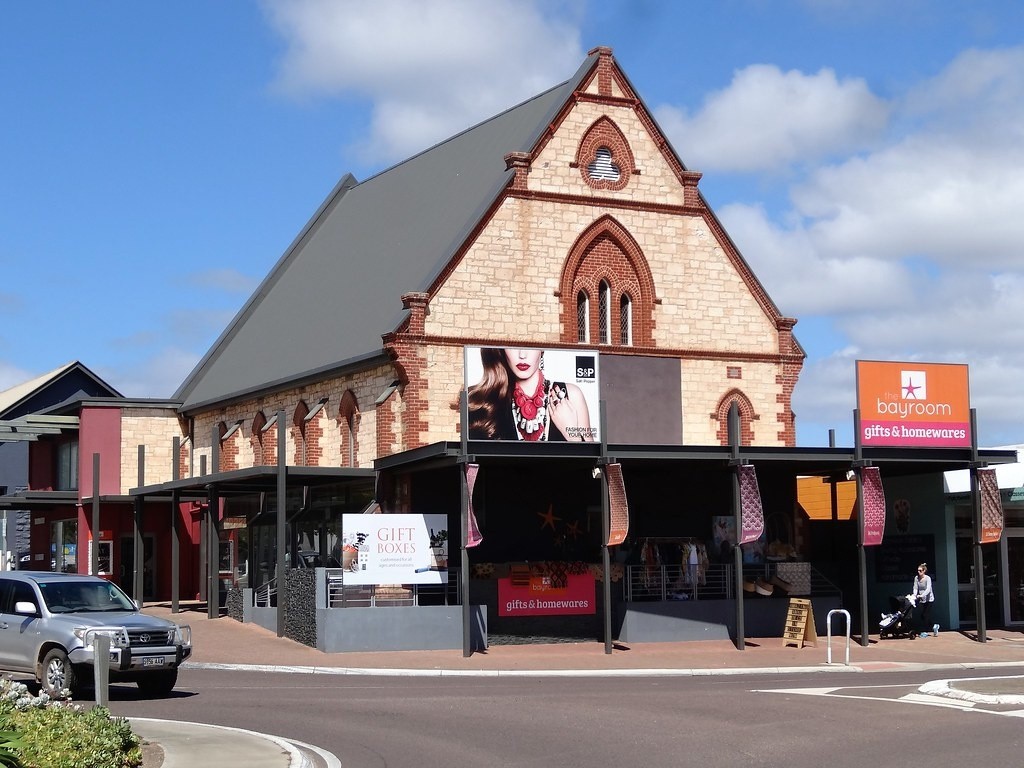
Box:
[877,593,919,641]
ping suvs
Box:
[0,571,193,701]
[274,551,343,590]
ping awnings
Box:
[303,398,329,420]
[374,380,400,403]
[178,433,191,448]
[221,420,244,440]
[261,415,277,431]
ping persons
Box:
[881,611,902,621]
[912,563,940,639]
[717,539,735,565]
[467,348,594,442]
[767,530,796,557]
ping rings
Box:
[554,400,559,406]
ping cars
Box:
[239,547,269,575]
[52,553,70,570]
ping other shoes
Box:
[881,613,887,619]
[933,624,940,636]
[919,632,928,639]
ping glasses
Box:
[917,570,923,573]
